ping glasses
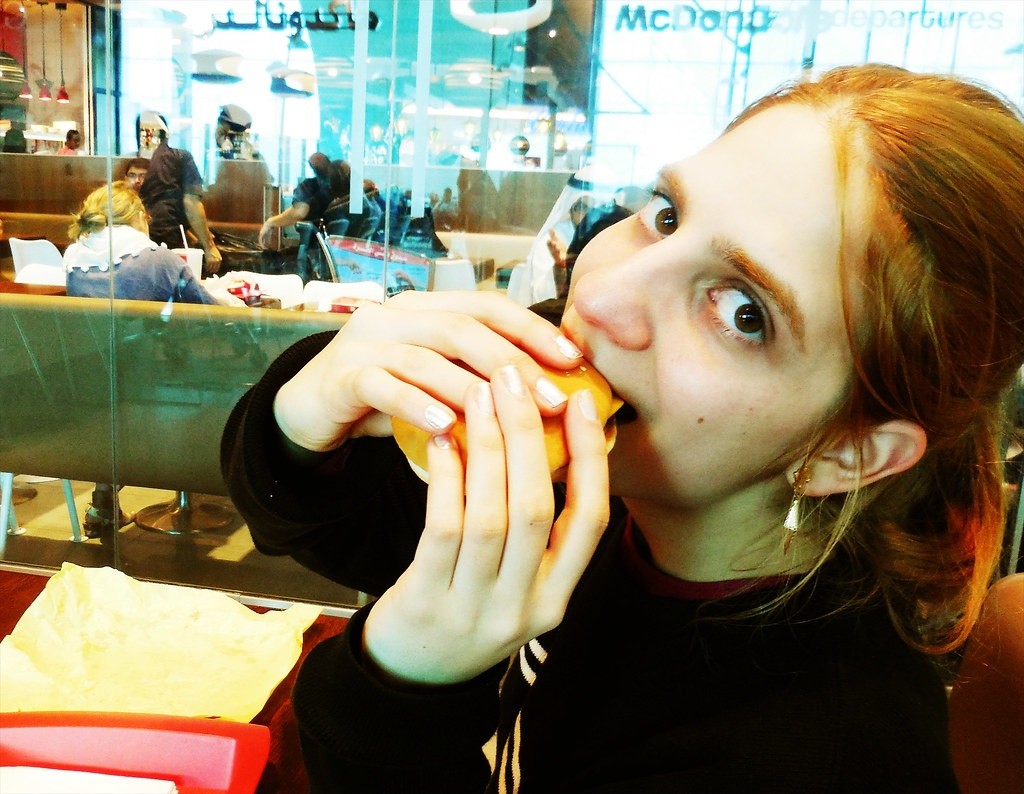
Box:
[137,210,153,225]
[126,173,145,180]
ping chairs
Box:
[7,237,65,286]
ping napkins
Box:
[0,561,326,727]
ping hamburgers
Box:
[389,355,619,487]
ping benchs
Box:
[363,161,576,280]
[0,152,281,254]
[0,291,355,535]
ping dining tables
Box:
[0,561,362,794]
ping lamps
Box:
[18,0,70,105]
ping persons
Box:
[258,152,330,249]
[327,160,375,207]
[221,68,1023,794]
[57,130,85,155]
[3,128,27,153]
[125,159,149,190]
[63,180,219,305]
[137,111,273,274]
